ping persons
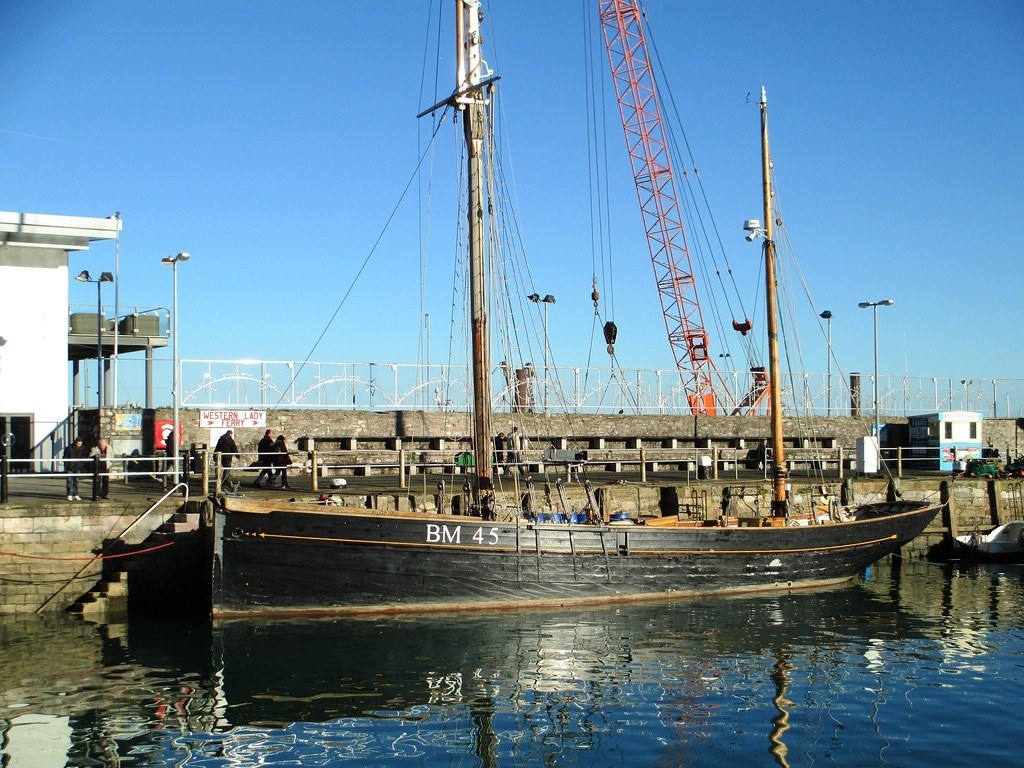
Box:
[64,437,88,501]
[213,430,241,485]
[972,525,982,547]
[757,439,770,470]
[156,427,182,483]
[89,438,114,499]
[499,426,529,477]
[253,429,293,489]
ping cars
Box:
[746,450,772,470]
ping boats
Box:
[956,521,1024,564]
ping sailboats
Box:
[209,586,949,768]
[200,0,968,627]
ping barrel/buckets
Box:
[610,514,629,521]
[530,513,587,525]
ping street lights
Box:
[961,380,973,410]
[75,271,114,408]
[858,299,894,470]
[162,253,190,494]
[527,293,556,414]
[719,354,731,415]
[820,311,832,417]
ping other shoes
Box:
[102,495,108,499]
[93,496,100,500]
[68,495,72,501]
[282,485,290,489]
[74,495,82,500]
[266,480,271,488]
[254,482,261,486]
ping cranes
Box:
[583,0,772,415]
